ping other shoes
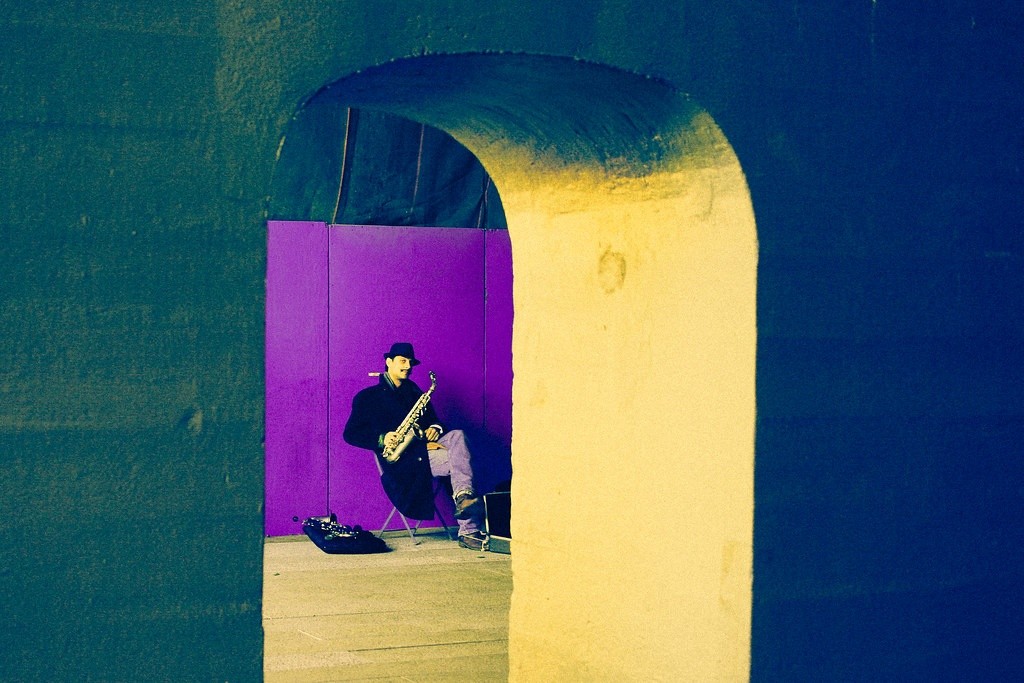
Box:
[454,493,483,520]
[459,532,489,550]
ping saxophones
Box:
[301,511,361,542]
[381,369,439,466]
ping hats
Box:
[384,343,421,367]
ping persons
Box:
[344,341,489,550]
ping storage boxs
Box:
[483,491,511,555]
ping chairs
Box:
[371,452,454,545]
